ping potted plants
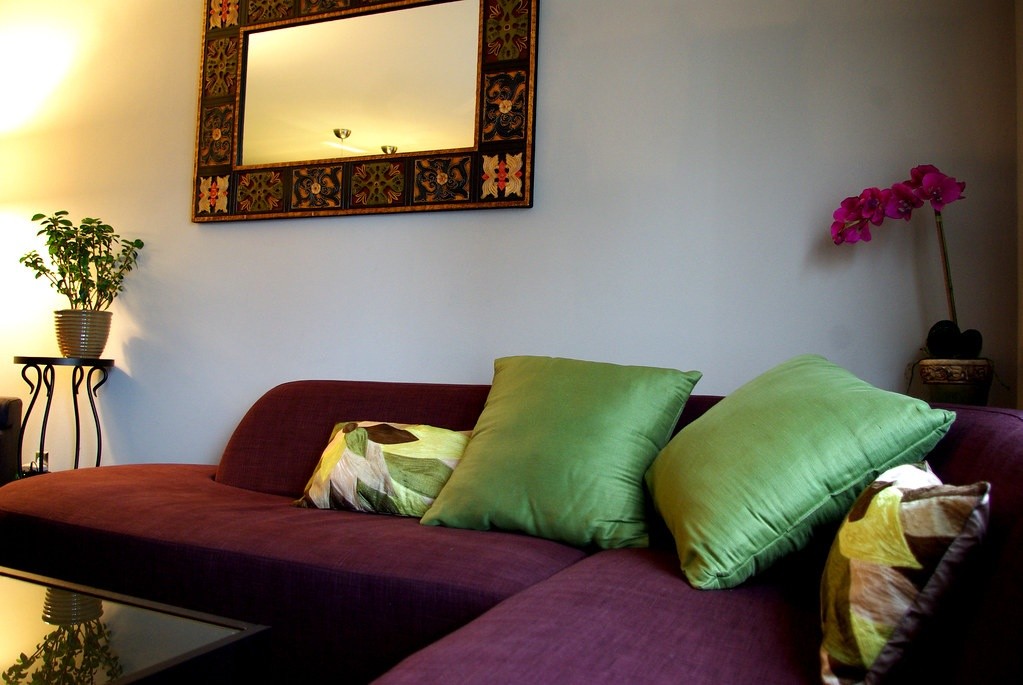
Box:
[19,211,144,359]
[2,588,123,685]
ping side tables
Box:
[13,356,114,479]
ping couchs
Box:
[0,380,1023,685]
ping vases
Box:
[919,359,994,406]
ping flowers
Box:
[830,165,968,320]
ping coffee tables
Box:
[0,566,265,685]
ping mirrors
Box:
[187,0,541,222]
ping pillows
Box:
[293,422,477,517]
[420,356,704,551]
[819,457,994,685]
[645,355,957,590]
